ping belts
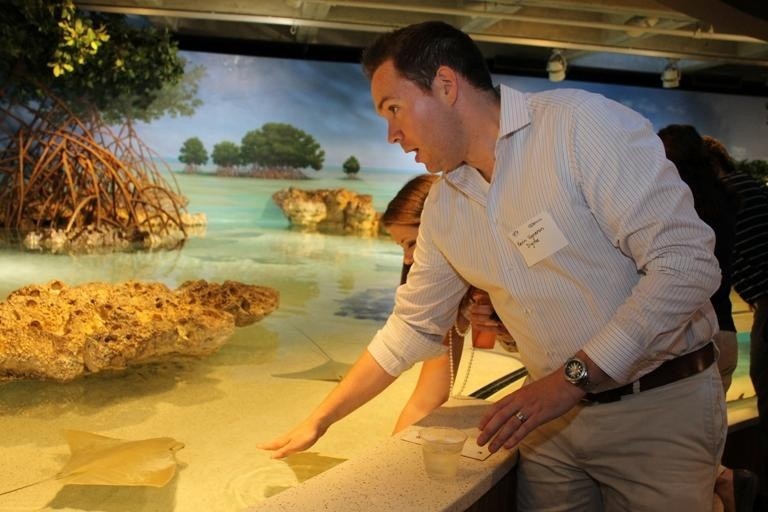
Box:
[564,341,718,404]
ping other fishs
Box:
[0,428,185,495]
[271,332,353,382]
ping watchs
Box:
[563,356,594,394]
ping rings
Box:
[516,411,528,423]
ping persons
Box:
[378,172,519,435]
[257,23,727,511]
[701,136,768,511]
[653,125,756,510]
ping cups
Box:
[417,423,466,481]
[471,319,498,350]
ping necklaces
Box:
[449,312,475,399]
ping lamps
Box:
[545,45,683,92]
